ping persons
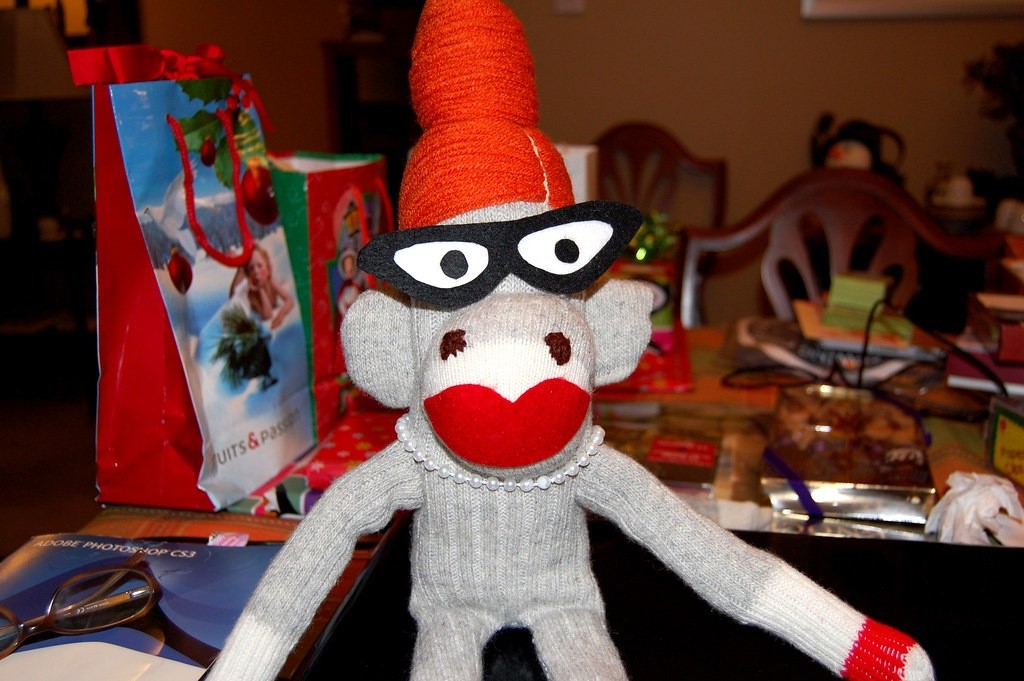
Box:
[337,249,363,384]
[245,244,295,330]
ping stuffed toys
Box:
[205,5,934,681]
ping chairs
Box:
[590,121,729,231]
[677,167,1004,337]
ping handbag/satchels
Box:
[761,381,937,524]
[66,44,316,511]
[272,151,396,446]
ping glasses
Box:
[0,550,164,659]
